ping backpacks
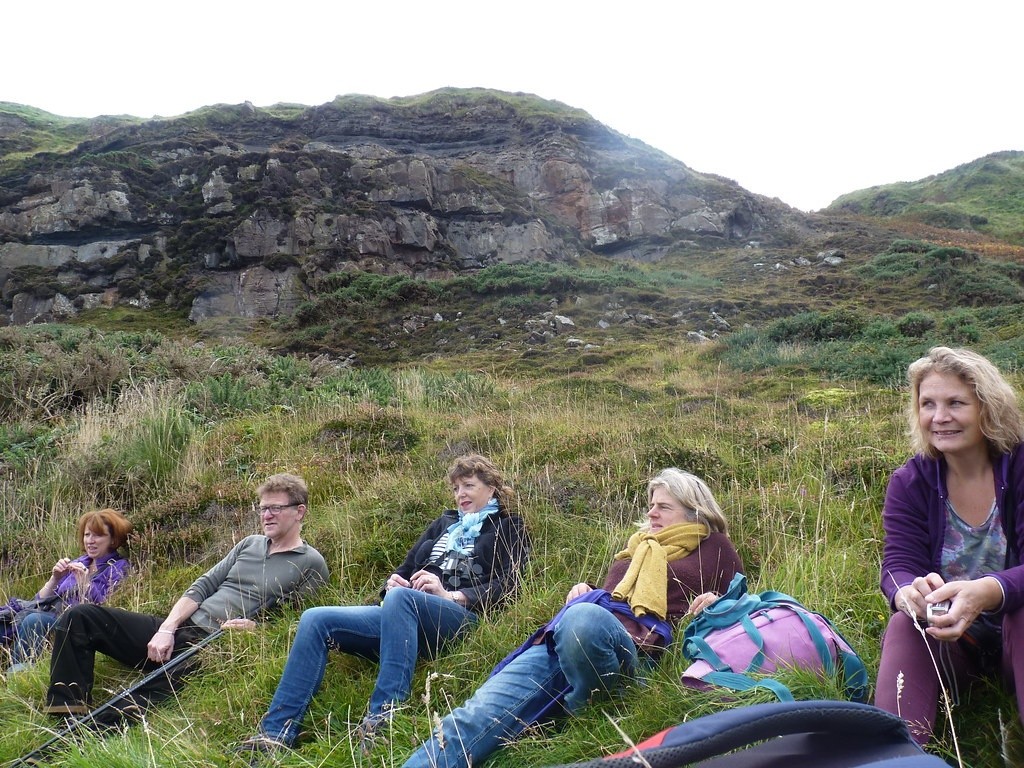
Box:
[558,700,952,768]
[681,572,867,703]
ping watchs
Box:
[449,591,460,605]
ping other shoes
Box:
[43,688,92,714]
[242,737,286,752]
[25,749,58,768]
[361,716,386,751]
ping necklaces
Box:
[92,567,95,572]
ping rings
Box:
[83,567,86,572]
[899,601,906,608]
[959,616,968,622]
[428,578,432,584]
[706,598,712,605]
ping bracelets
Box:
[44,582,58,590]
[157,630,175,635]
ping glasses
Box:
[255,502,303,517]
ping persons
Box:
[6,508,136,678]
[225,456,531,768]
[400,467,747,768]
[874,347,1024,750]
[25,473,331,768]
